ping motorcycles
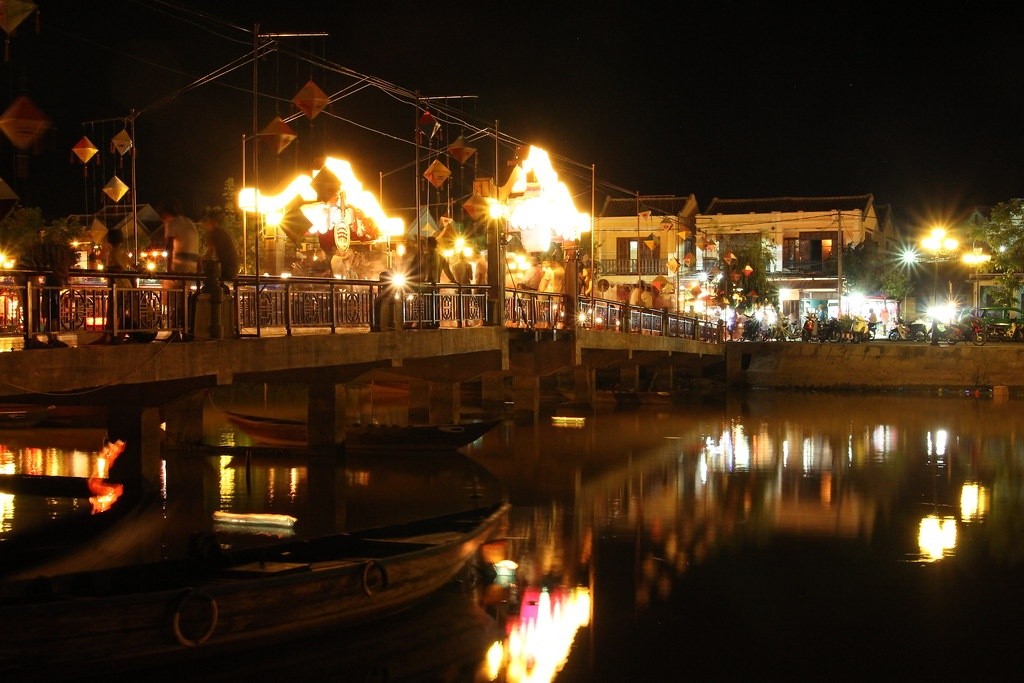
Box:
[888,317,930,342]
[943,317,1024,346]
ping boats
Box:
[222,409,504,458]
[0,498,525,680]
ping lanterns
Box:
[637,204,757,308]
[0,0,496,227]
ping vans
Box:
[967,304,1024,342]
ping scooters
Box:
[741,316,880,344]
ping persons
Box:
[163,206,201,341]
[90,228,144,345]
[629,280,890,338]
[201,213,241,295]
[372,234,592,329]
[13,240,72,349]
[957,308,980,339]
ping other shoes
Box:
[24,335,54,349]
[47,339,68,347]
